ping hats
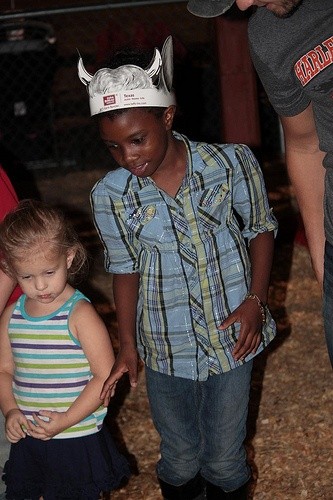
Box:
[77,35,176,117]
[186,0,235,17]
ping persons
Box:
[184,0,333,377]
[0,168,25,318]
[0,199,122,499]
[72,47,282,498]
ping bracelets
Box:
[242,294,267,327]
